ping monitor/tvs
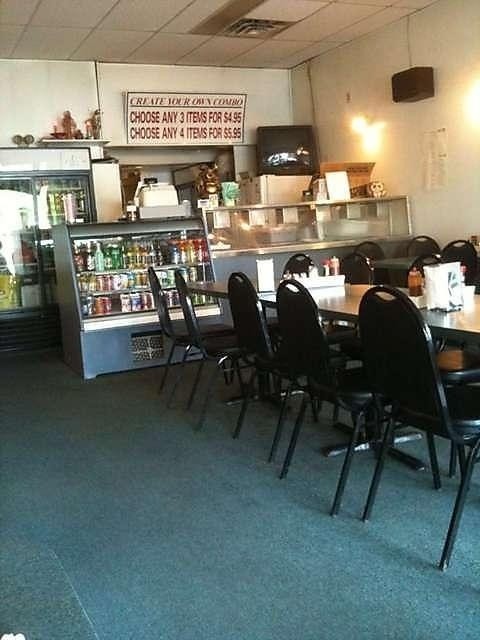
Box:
[257,125,316,175]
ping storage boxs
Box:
[320,161,376,198]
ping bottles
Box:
[126,205,138,223]
[324,266,329,277]
[283,265,318,280]
[41,178,88,309]
[330,256,340,275]
[407,266,423,297]
[73,236,218,317]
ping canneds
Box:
[86,268,214,315]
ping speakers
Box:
[392,66,434,102]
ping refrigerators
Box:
[0,146,98,359]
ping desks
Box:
[372,255,480,283]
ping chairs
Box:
[338,253,376,281]
[436,239,478,272]
[224,271,341,464]
[401,235,441,253]
[274,278,443,517]
[353,242,387,262]
[144,267,235,408]
[282,250,312,273]
[408,253,451,278]
[354,282,480,573]
[172,270,282,433]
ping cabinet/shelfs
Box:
[199,194,414,282]
[50,217,223,381]
